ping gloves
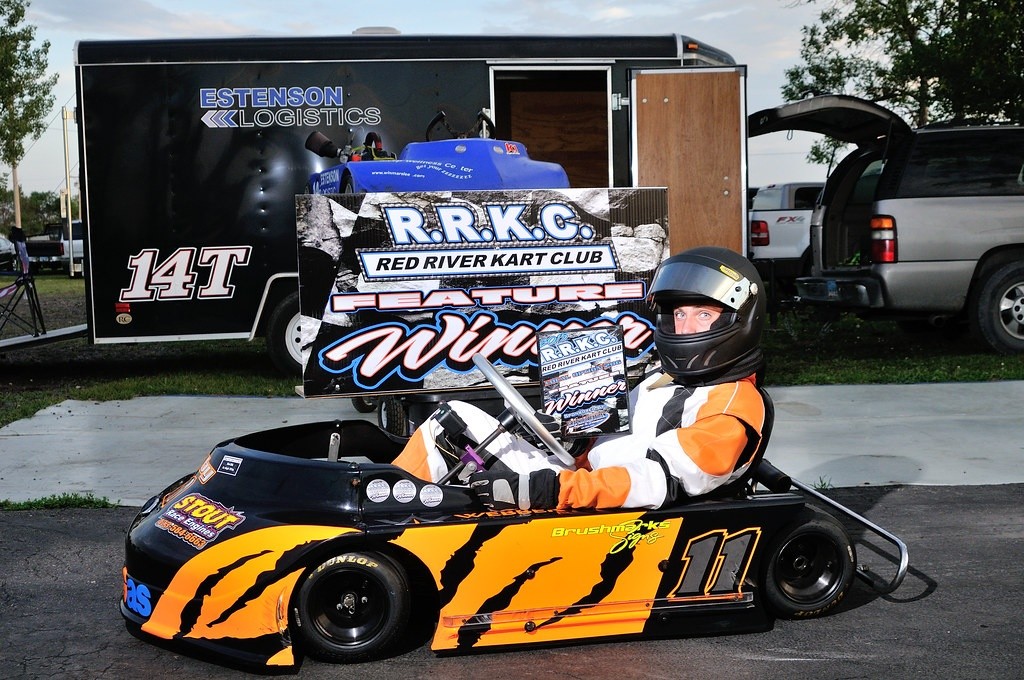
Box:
[528,408,561,449]
[468,467,557,511]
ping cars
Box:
[0,237,20,273]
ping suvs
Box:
[26,220,82,278]
[749,92,1024,352]
[748,182,825,309]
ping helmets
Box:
[644,245,768,375]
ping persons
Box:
[390,245,772,512]
[7,225,29,272]
